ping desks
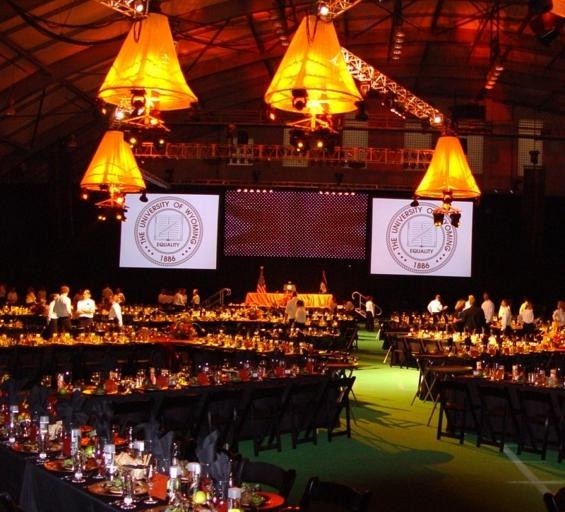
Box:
[0,303,368,512]
[245,292,333,308]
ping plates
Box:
[0,326,358,398]
[0,401,286,512]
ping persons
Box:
[365,296,376,330]
[294,300,305,328]
[285,291,299,325]
[0,282,126,336]
[552,300,565,323]
[159,285,201,313]
[454,292,536,334]
[427,294,449,324]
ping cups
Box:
[0,401,243,512]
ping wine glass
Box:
[1,405,215,512]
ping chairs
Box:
[377,312,565,464]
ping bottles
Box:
[476,357,565,388]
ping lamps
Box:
[96,13,198,132]
[411,136,481,228]
[263,14,363,132]
[78,130,147,222]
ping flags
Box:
[256,267,266,294]
[319,270,328,294]
